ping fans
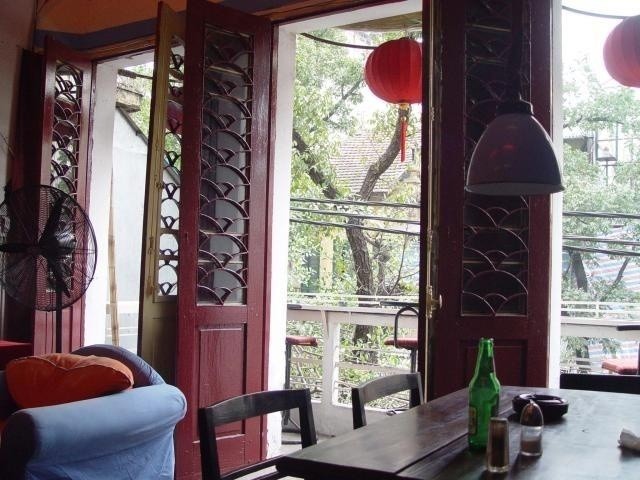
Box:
[2,184,98,354]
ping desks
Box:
[277,384,640,479]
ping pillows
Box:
[8,350,136,408]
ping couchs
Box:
[2,345,187,480]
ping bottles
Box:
[486,416,510,474]
[468,337,501,455]
[520,398,544,457]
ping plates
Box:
[512,393,569,418]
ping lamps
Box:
[462,0,565,193]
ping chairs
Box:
[385,308,419,414]
[352,372,423,429]
[602,324,640,375]
[282,334,320,444]
[198,388,317,480]
